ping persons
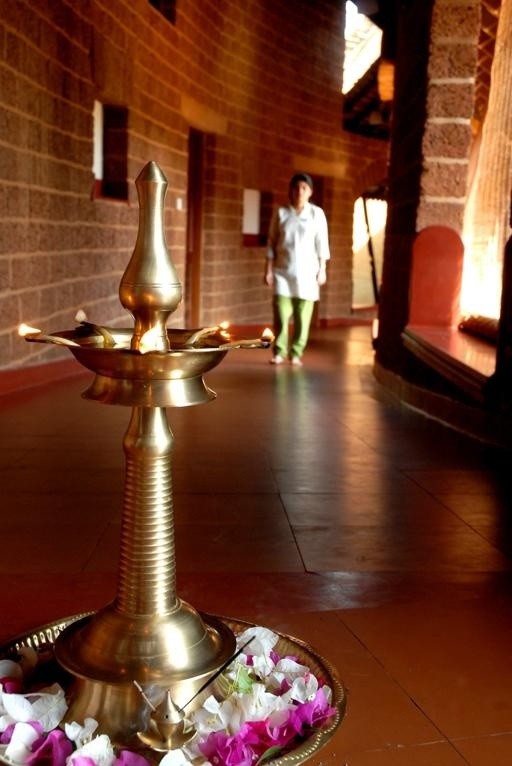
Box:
[263,171,331,366]
